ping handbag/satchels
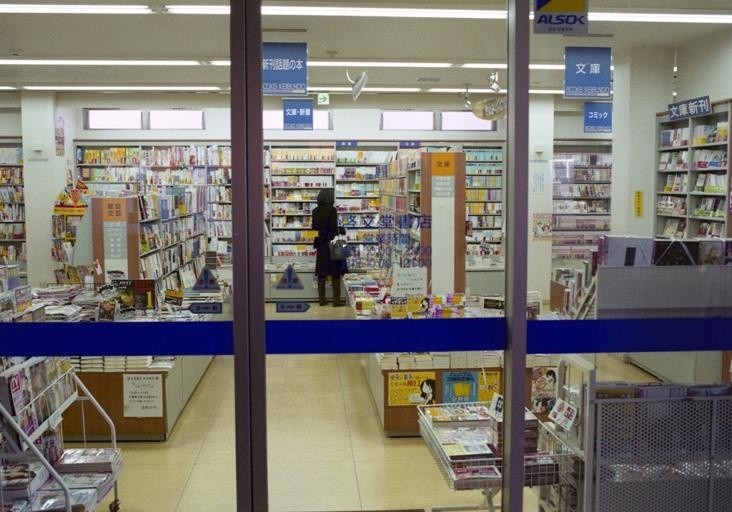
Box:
[329,240,351,260]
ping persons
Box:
[311,189,345,308]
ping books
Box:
[261,146,422,301]
[536,381,731,511]
[551,235,730,321]
[464,148,505,255]
[376,350,567,490]
[0,145,231,322]
[417,291,541,318]
[0,357,177,512]
[346,272,416,319]
[551,151,613,262]
[655,122,725,238]
[423,146,452,153]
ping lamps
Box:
[345,67,369,102]
[458,83,472,107]
[488,72,501,91]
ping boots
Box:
[319,282,327,305]
[332,279,345,306]
